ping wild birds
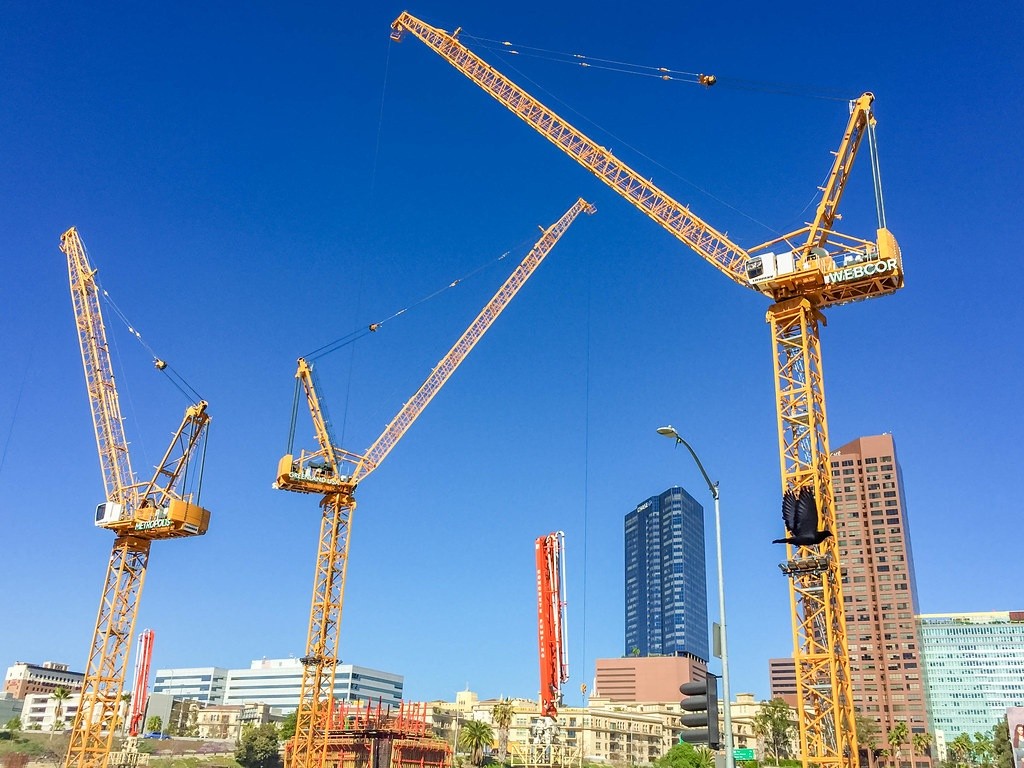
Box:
[770,486,834,548]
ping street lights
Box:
[656,425,735,768]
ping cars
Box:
[144,731,172,740]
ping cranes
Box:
[273,197,598,768]
[390,9,905,768]
[58,226,212,768]
